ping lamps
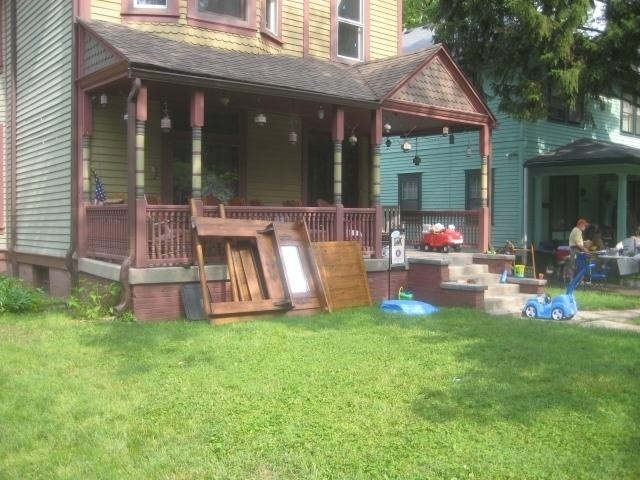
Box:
[347,112,362,146]
[384,111,474,166]
[157,87,173,133]
[217,92,325,147]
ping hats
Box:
[577,219,591,226]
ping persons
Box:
[570,218,640,286]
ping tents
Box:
[94,173,108,205]
